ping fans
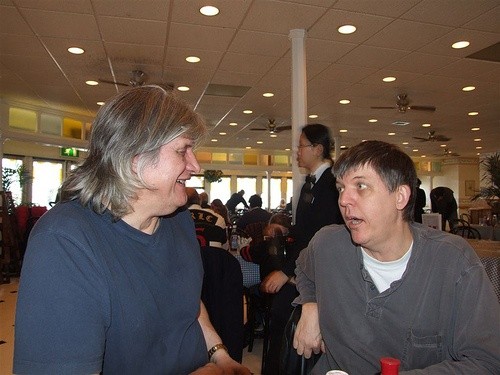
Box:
[412,130,449,142]
[370,93,436,113]
[98,69,175,92]
[249,118,292,133]
[432,149,459,157]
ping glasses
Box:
[297,144,317,151]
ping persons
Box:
[259,123,346,374]
[291,139,500,375]
[431,186,459,234]
[14,84,254,375]
[414,178,427,225]
[182,187,292,329]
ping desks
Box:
[465,237,500,301]
[231,251,272,288]
[469,206,491,224]
[454,224,500,240]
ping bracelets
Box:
[208,343,228,360]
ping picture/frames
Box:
[464,179,475,196]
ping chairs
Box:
[228,206,248,240]
[200,246,242,362]
[449,213,481,240]
